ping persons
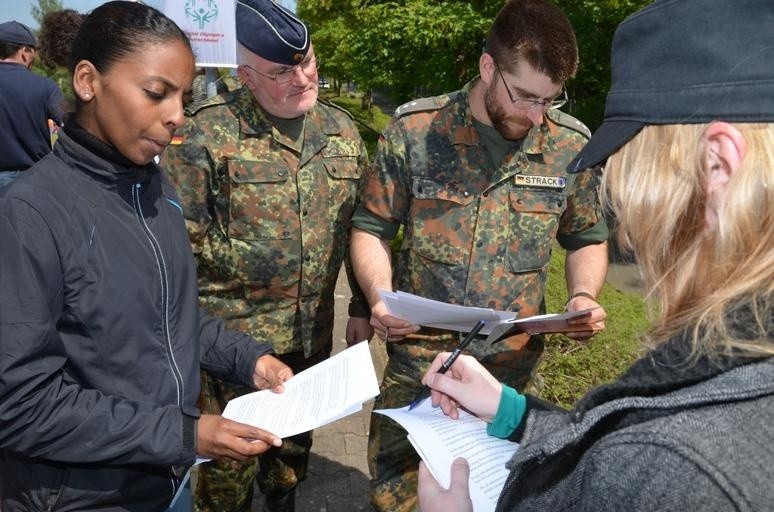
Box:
[0,1,297,512]
[0,20,68,193]
[417,1,773,512]
[351,1,610,512]
[156,2,371,512]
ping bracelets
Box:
[562,292,599,304]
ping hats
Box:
[0,20,41,50]
[566,1,774,174]
[236,0,310,65]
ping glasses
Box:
[492,62,568,110]
[242,57,321,83]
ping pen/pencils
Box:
[386,326,389,342]
[408,320,486,412]
